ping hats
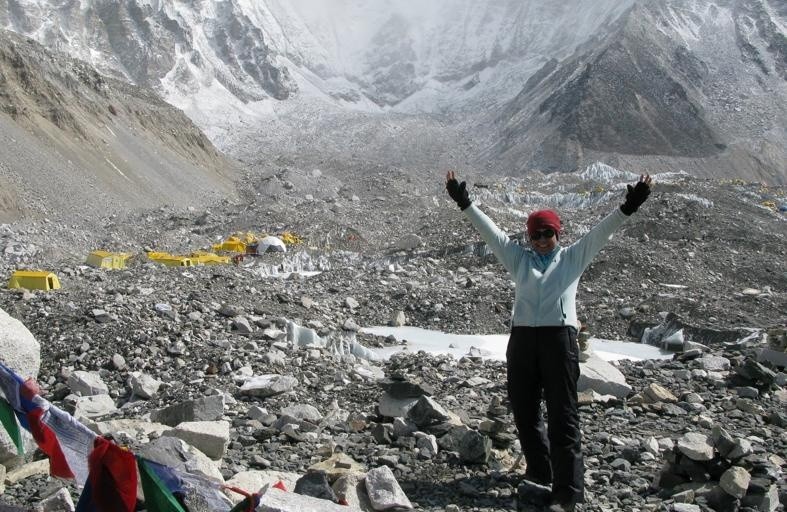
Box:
[526,210,561,238]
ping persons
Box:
[442,168,654,506]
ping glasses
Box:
[529,229,556,241]
